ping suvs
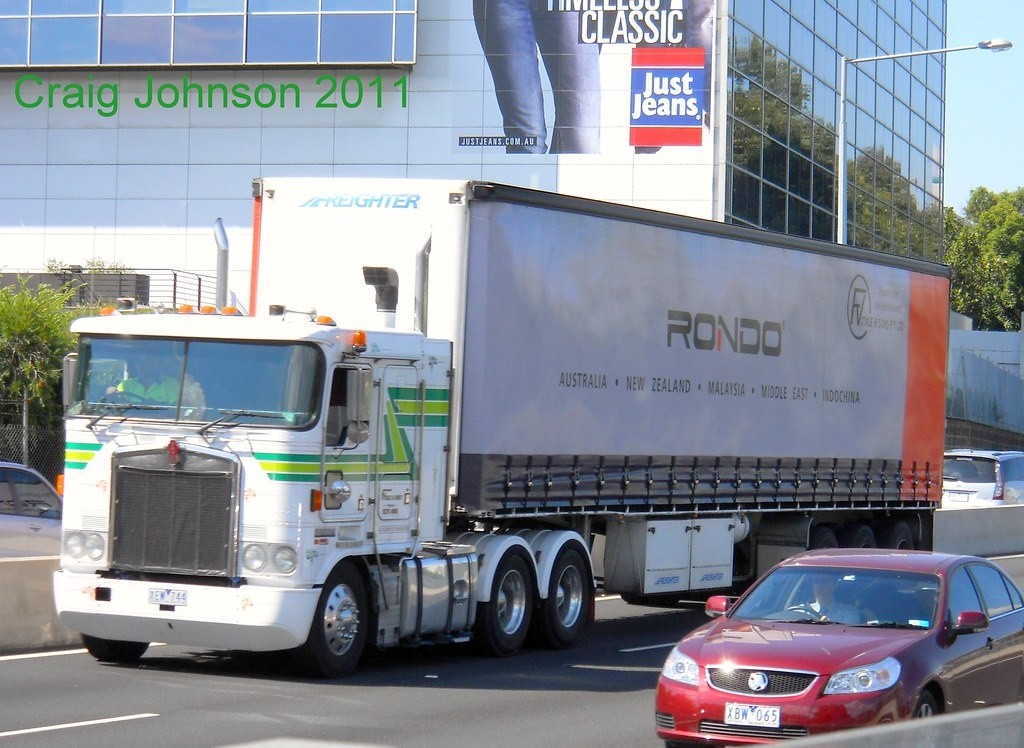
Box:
[938,449,1024,508]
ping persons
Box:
[785,572,859,627]
[106,347,183,410]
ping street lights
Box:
[830,40,1017,241]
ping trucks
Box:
[55,177,953,674]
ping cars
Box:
[647,544,1024,748]
[0,461,65,560]
